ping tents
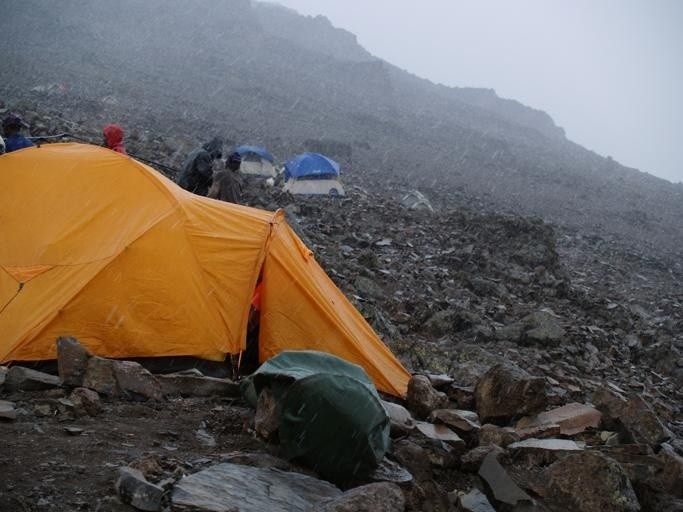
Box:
[280,151,349,201]
[231,143,276,179]
[0,141,418,402]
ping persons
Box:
[101,125,127,154]
[173,135,223,196]
[0,110,36,152]
[205,151,247,207]
